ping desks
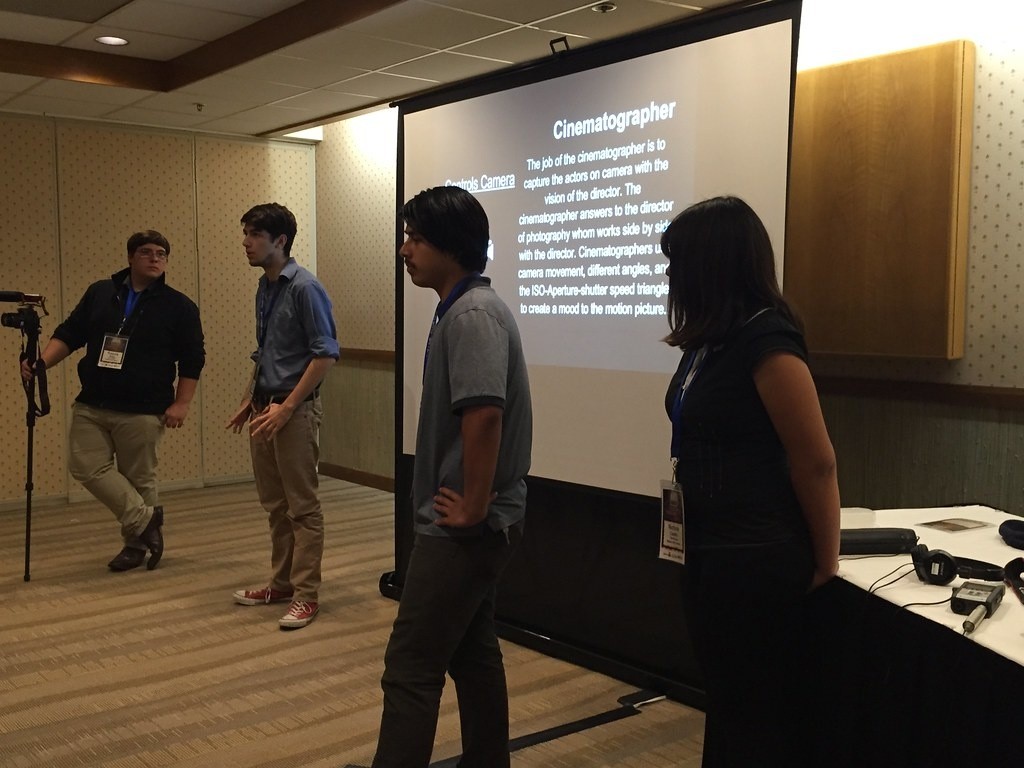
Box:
[835,504,1024,768]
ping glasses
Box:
[134,248,168,259]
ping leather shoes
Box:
[139,505,164,570]
[108,546,146,571]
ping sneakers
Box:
[232,583,294,606]
[278,601,320,628]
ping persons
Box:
[660,196,840,768]
[344,185,533,768]
[20,230,206,571]
[225,202,340,628]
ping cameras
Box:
[1,308,40,331]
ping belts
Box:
[254,390,320,405]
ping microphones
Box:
[0,290,42,305]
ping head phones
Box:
[911,544,1006,585]
